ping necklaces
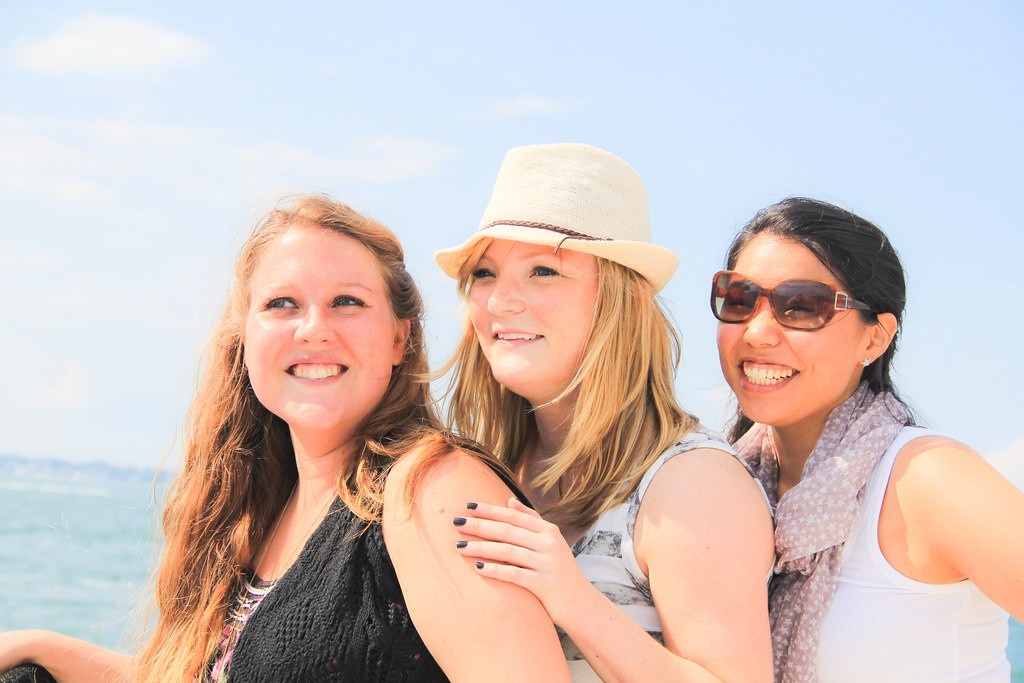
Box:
[216,478,336,683]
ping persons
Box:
[425,144,776,683]
[710,198,1024,683]
[0,194,571,683]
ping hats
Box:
[431,143,680,298]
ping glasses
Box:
[709,269,879,332]
